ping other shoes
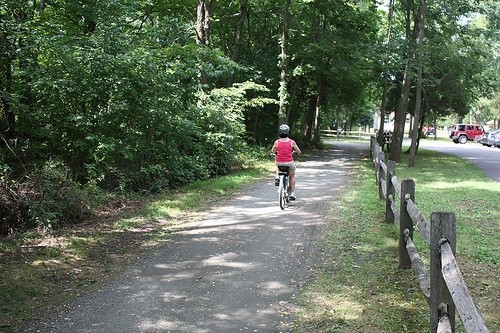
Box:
[275,177,280,186]
[289,195,296,201]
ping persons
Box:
[271,124,301,199]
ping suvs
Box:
[448,124,486,144]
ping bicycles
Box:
[270,150,302,210]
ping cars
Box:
[423,124,435,135]
[480,129,500,148]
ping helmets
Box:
[278,124,290,135]
